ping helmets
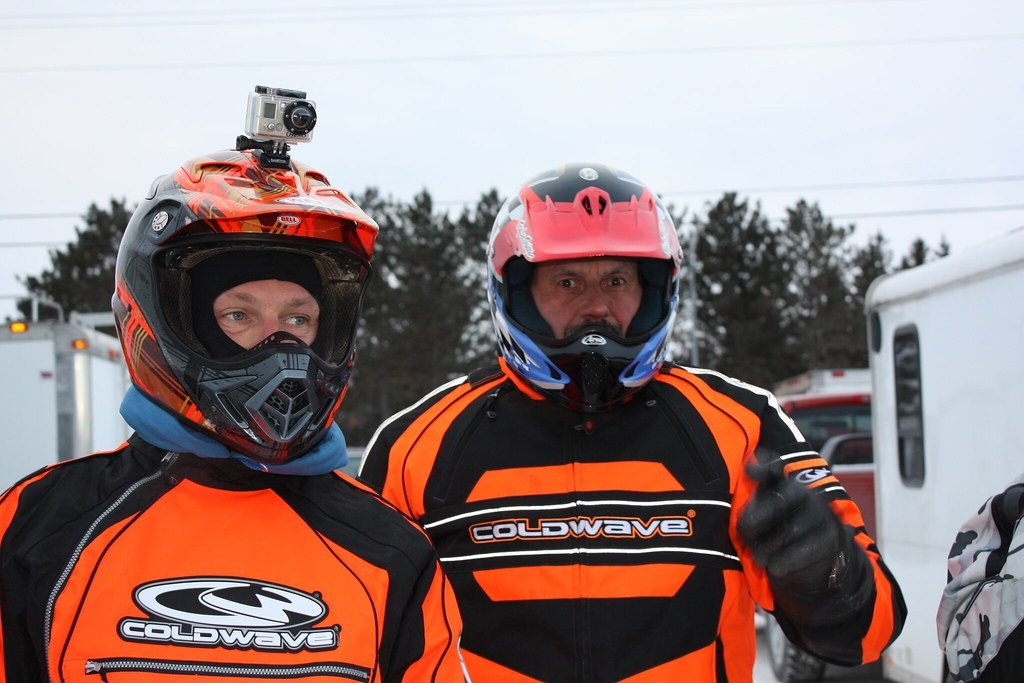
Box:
[112,142,383,468]
[484,160,685,432]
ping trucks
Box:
[769,225,1024,682]
[0,290,132,499]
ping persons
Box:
[933,465,1023,683]
[352,152,908,683]
[0,146,468,683]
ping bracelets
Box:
[823,542,852,591]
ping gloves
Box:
[736,450,845,597]
